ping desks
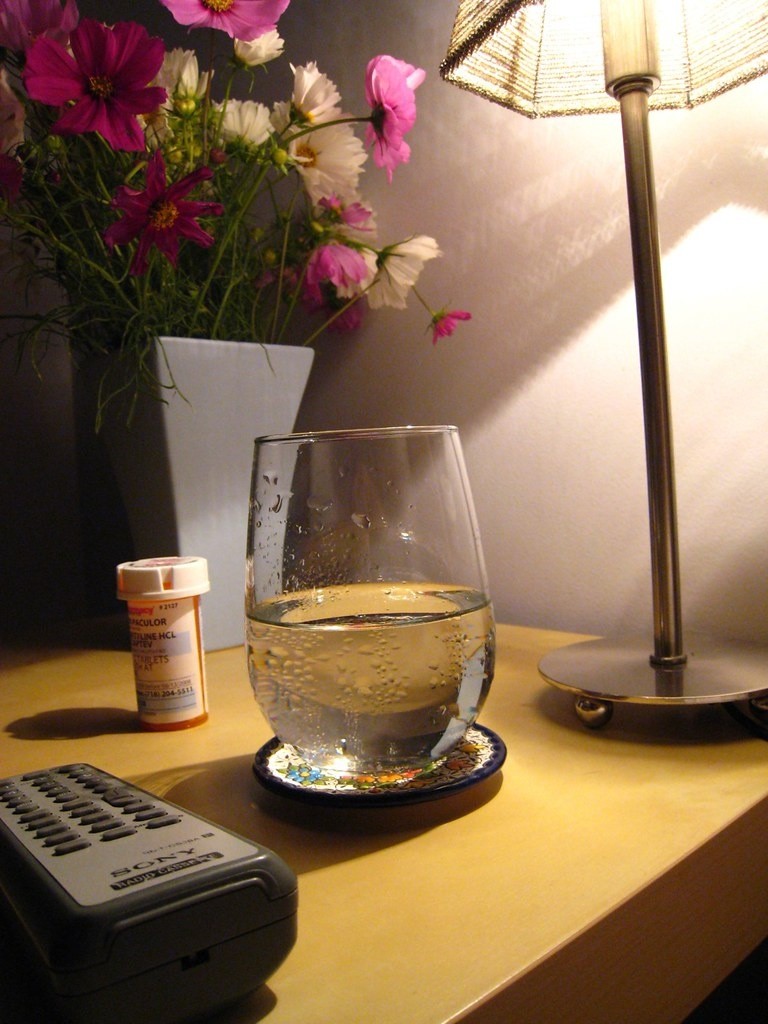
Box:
[0,622,767,1024]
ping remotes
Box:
[0,763,296,1024]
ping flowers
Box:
[0,1,471,432]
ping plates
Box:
[252,720,507,807]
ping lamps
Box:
[437,4,768,735]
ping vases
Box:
[113,336,317,655]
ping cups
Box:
[243,424,496,779]
